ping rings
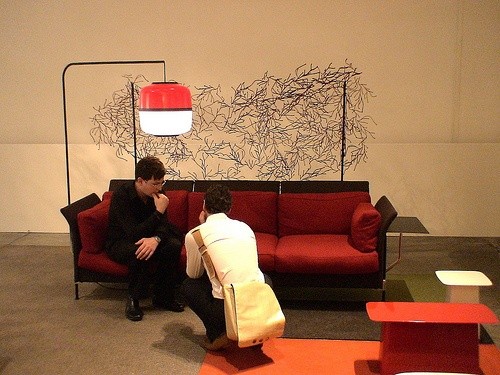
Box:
[159,193,163,197]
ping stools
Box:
[436,270,493,340]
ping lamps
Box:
[62,60,192,206]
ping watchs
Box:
[151,235,161,244]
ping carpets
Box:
[197,236,500,375]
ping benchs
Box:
[60,179,398,302]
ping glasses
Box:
[142,178,166,189]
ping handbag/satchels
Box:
[222,282,286,348]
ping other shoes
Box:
[200,334,230,351]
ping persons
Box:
[180,185,264,351]
[105,156,185,321]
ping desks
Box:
[386,216,429,272]
[365,302,500,375]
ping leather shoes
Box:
[125,297,143,320]
[168,295,184,312]
